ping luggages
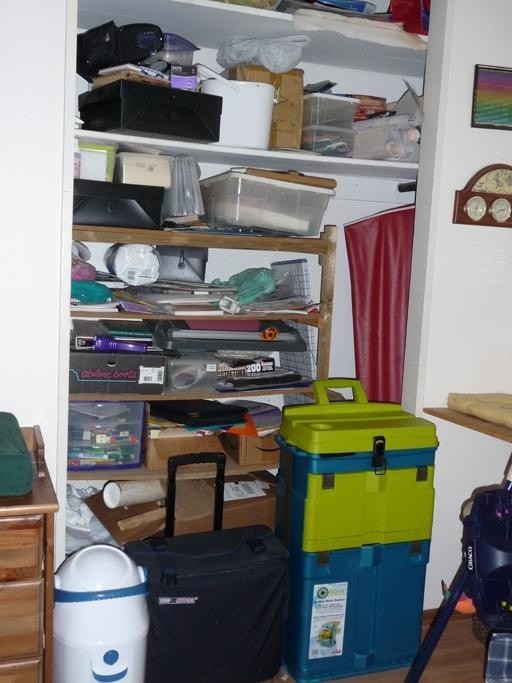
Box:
[124,452,290,683]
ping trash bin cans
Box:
[51,542,150,683]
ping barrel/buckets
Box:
[198,62,275,149]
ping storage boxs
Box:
[68,401,146,472]
[143,402,230,470]
[218,401,286,465]
[276,377,438,680]
[117,152,173,188]
[72,66,387,165]
[71,318,221,394]
[197,167,336,237]
[72,137,117,182]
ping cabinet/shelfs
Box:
[0,426,60,682]
[58,1,457,572]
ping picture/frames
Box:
[470,64,512,131]
